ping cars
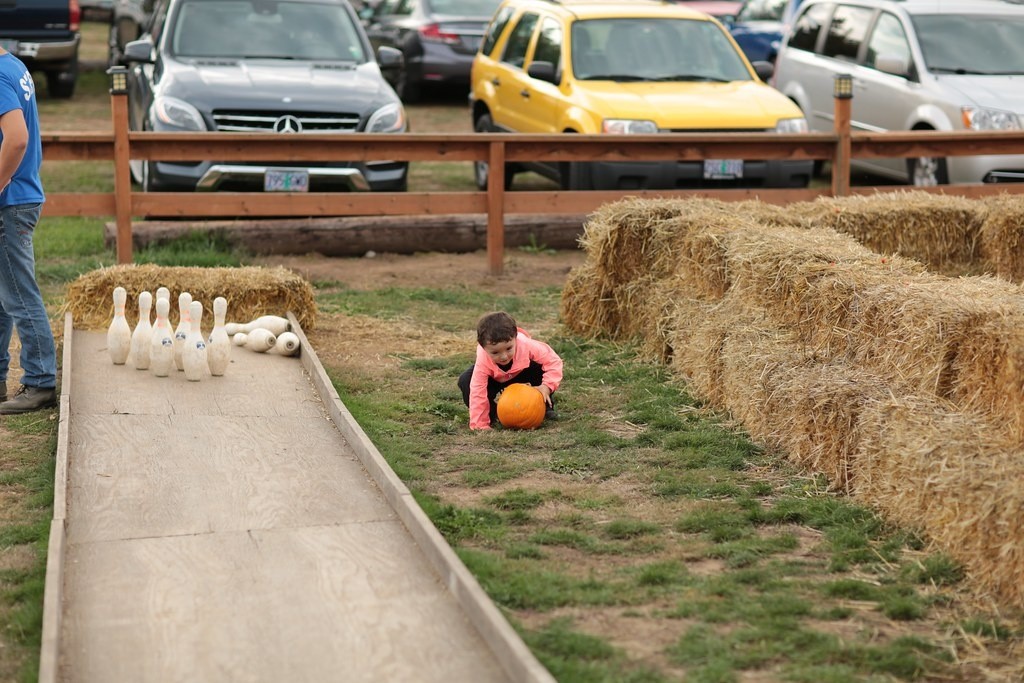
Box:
[122,0,411,221]
[0,0,82,99]
[358,0,804,104]
[469,0,814,191]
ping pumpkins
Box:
[497,383,546,430]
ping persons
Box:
[456,312,563,430]
[0,45,60,416]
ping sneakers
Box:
[0,383,58,413]
[0,380,8,401]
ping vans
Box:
[771,0,1024,187]
